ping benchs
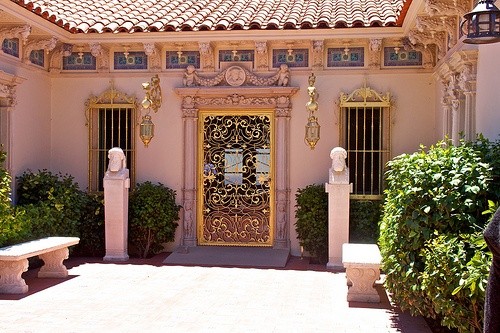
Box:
[0,237,80,294]
[342,242,384,302]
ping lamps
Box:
[140,74,162,148]
[460,0,500,44]
[305,73,320,150]
